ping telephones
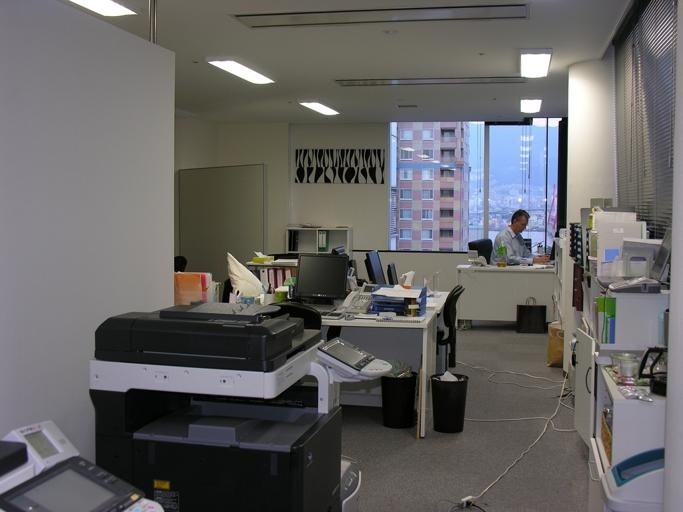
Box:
[343,292,359,308]
[610,277,659,294]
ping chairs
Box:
[468,239,493,265]
[435,285,464,367]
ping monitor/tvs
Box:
[550,232,559,260]
[294,253,349,311]
[331,246,345,254]
[364,249,386,284]
[387,263,398,284]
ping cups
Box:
[619,358,640,385]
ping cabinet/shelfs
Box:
[284,227,352,263]
[552,236,670,512]
[88,344,342,512]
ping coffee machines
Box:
[637,308,669,396]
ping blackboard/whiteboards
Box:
[179,163,267,282]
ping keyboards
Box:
[320,310,344,320]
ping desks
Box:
[455,264,557,330]
[230,289,450,439]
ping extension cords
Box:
[461,496,473,508]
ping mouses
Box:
[345,314,354,321]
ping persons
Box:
[487,209,551,268]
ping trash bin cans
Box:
[431,375,470,434]
[380,372,418,429]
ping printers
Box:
[88,300,393,512]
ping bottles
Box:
[497,241,508,269]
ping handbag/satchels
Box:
[517,297,546,333]
[547,321,565,367]
